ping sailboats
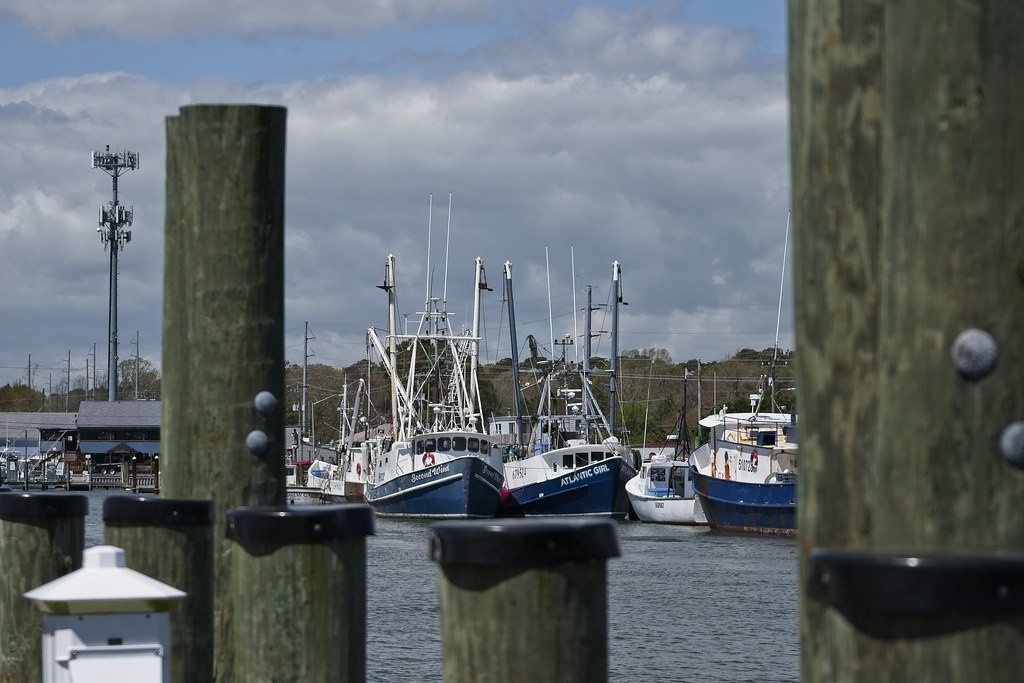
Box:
[0,189,798,536]
